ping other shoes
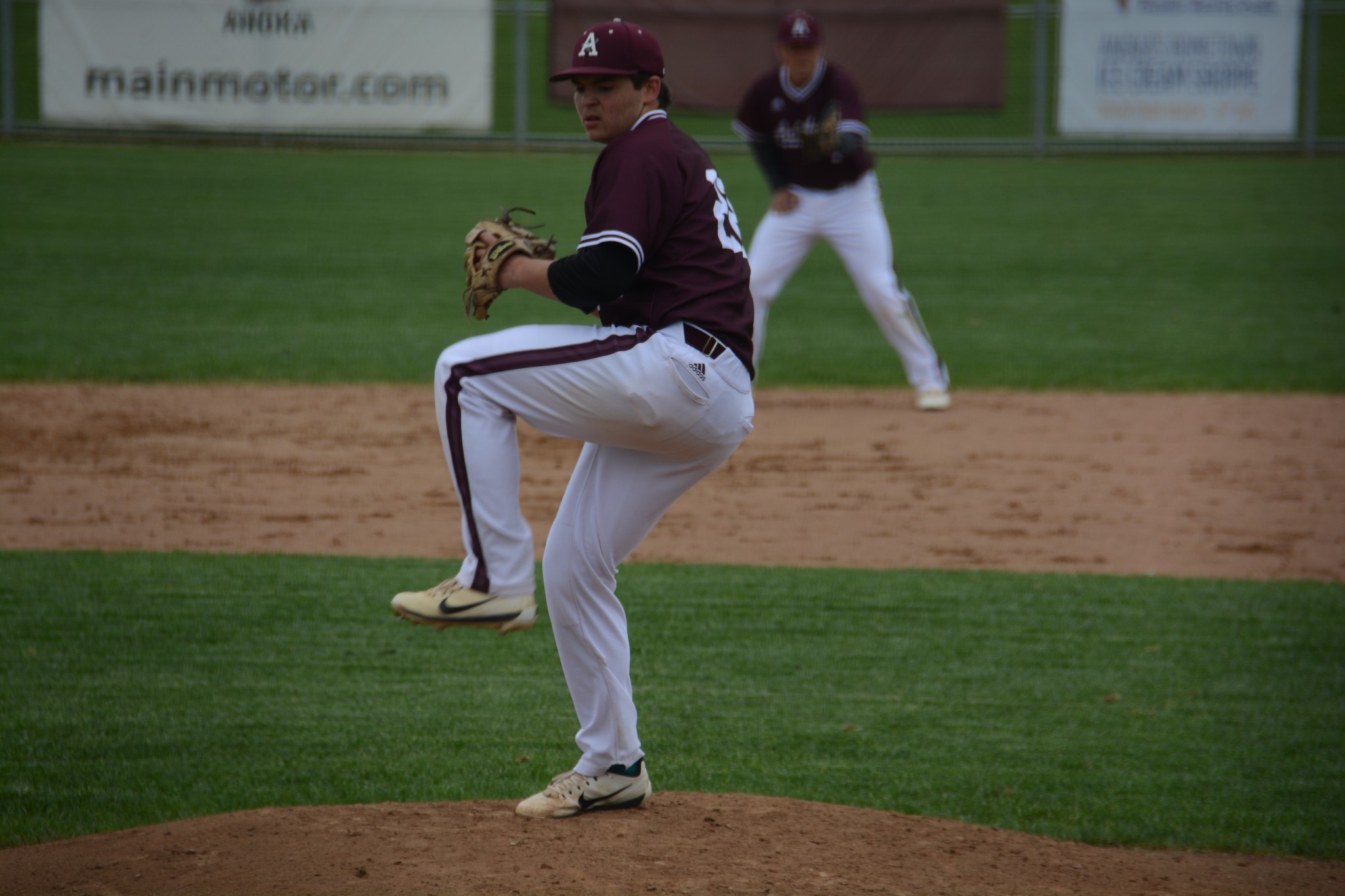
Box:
[915,388,952,412]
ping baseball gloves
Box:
[464,213,557,321]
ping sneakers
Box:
[514,758,653,821]
[388,579,537,633]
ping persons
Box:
[390,19,758,820]
[725,9,955,410]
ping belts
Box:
[679,320,727,362]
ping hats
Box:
[777,14,821,51]
[550,16,665,83]
